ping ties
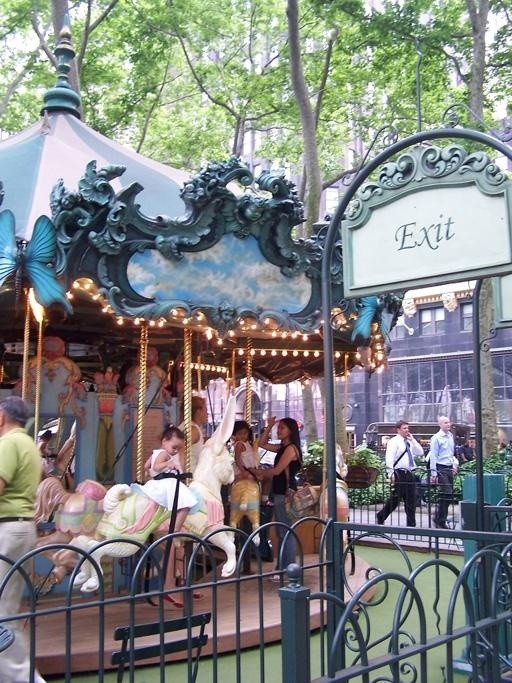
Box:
[404,438,414,466]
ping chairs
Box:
[110,610,212,681]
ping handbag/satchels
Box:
[285,482,319,520]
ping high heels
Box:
[163,592,203,609]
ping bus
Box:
[362,422,470,466]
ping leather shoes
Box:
[377,513,384,524]
[435,518,450,529]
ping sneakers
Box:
[269,570,287,583]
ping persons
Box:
[0,395,47,682]
[375,420,424,526]
[498,442,508,460]
[143,395,302,609]
[460,439,474,462]
[430,415,455,530]
[37,429,57,474]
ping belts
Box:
[1,517,31,521]
